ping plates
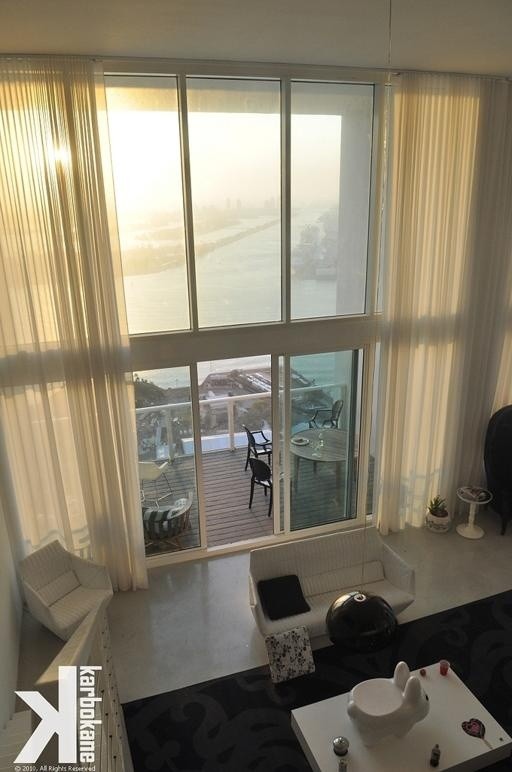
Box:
[291,437,310,447]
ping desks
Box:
[288,427,356,488]
[290,656,512,772]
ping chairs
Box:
[135,455,194,557]
[240,399,343,519]
[14,535,116,645]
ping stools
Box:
[456,484,493,538]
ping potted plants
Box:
[422,495,453,533]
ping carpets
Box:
[115,587,511,772]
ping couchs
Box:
[245,527,417,649]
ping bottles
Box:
[429,745,441,767]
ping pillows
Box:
[256,572,325,688]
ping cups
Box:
[439,661,450,676]
